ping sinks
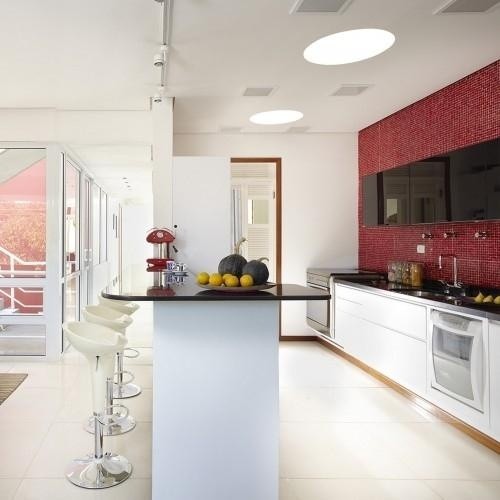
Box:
[387,287,446,296]
[419,296,457,302]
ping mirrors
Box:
[247,195,273,230]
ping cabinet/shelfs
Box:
[333,284,428,403]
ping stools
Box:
[61,320,132,490]
[83,302,137,436]
[97,289,143,401]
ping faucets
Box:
[438,253,463,289]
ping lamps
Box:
[151,0,171,104]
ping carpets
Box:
[0,373,29,405]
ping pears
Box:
[474,292,500,304]
[196,272,254,287]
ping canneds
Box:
[388,261,424,287]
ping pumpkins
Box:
[218,254,270,285]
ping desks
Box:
[101,277,332,500]
